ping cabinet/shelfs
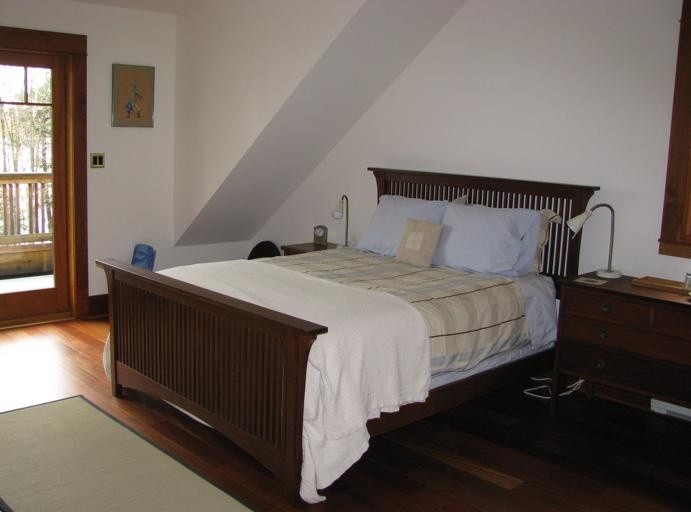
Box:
[548,267,691,424]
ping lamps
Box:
[330,195,352,249]
[566,202,623,280]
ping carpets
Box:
[0,393,257,512]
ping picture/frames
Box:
[109,63,155,128]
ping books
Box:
[651,398,691,421]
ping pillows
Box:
[354,193,562,280]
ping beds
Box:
[90,163,600,506]
